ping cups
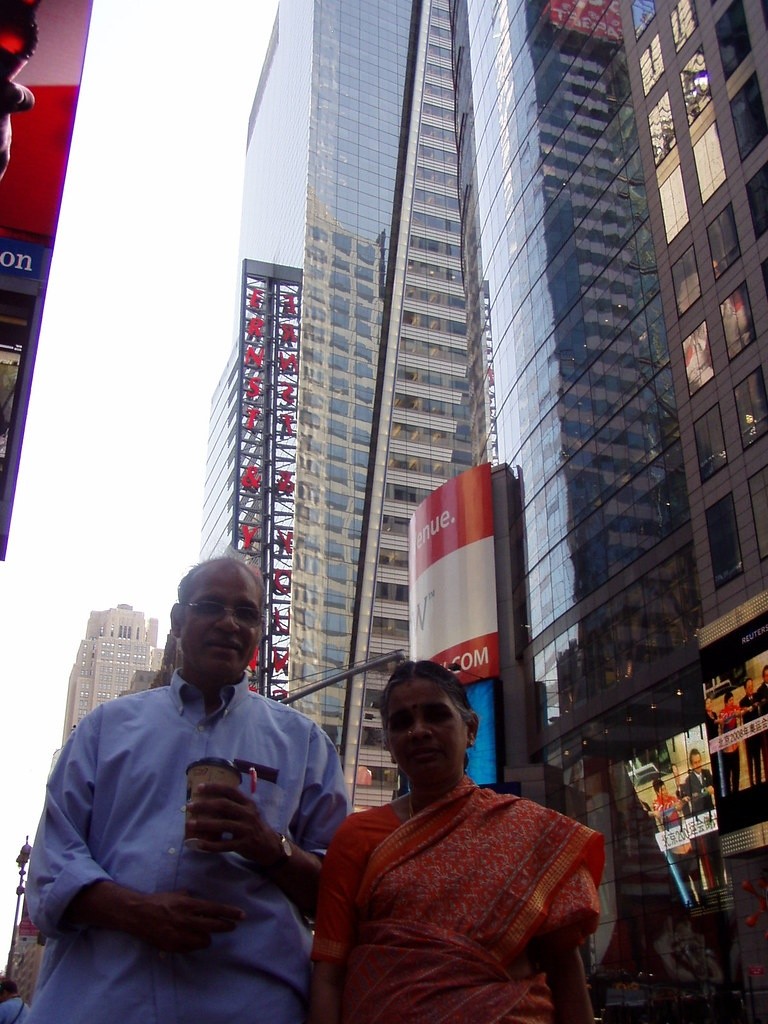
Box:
[183,757,242,854]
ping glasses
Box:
[182,600,266,627]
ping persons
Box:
[24,557,353,1024]
[705,697,724,799]
[0,981,29,1024]
[720,693,752,793]
[686,748,726,888]
[739,665,768,786]
[652,765,706,908]
[309,662,605,1024]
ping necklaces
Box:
[409,793,414,819]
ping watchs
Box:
[272,832,292,871]
